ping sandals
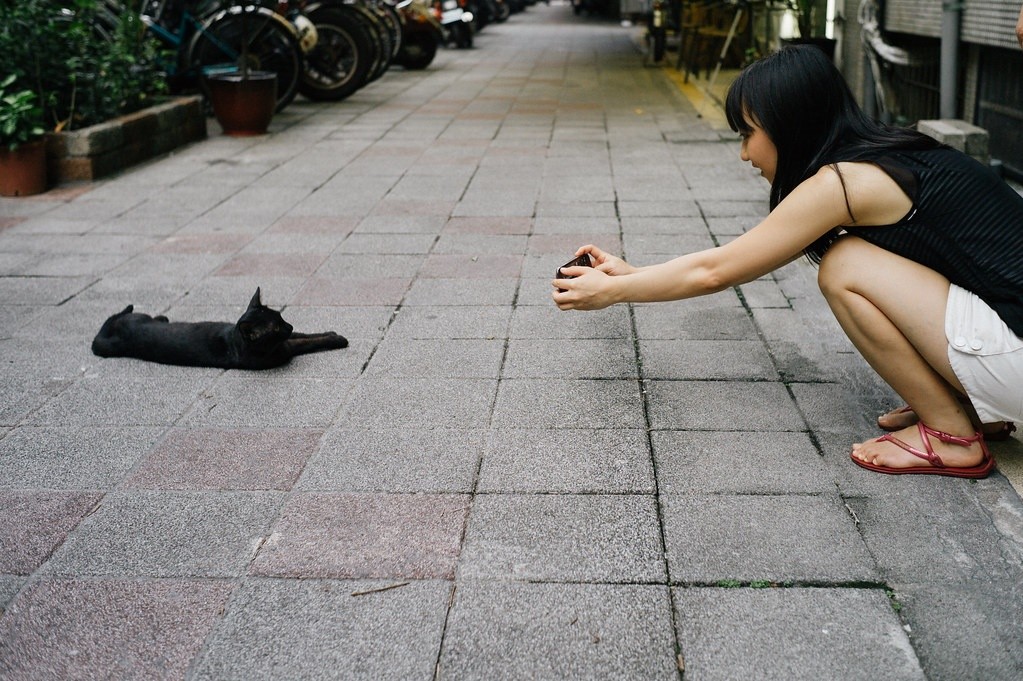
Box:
[850,421,997,478]
[877,395,1016,442]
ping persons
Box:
[551,46,1023,478]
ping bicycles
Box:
[19,0,535,112]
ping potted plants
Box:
[0,0,206,197]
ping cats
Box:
[89,288,349,371]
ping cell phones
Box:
[556,253,592,292]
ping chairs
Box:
[644,0,753,84]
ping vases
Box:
[207,71,277,136]
